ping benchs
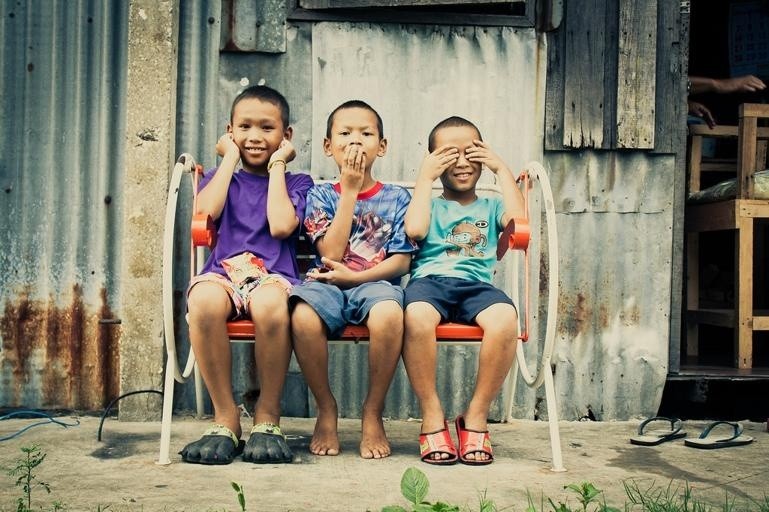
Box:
[155,153,565,473]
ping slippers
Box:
[684,421,753,449]
[419,420,459,466]
[455,415,494,466]
[178,424,247,465]
[630,417,687,446]
[242,422,294,465]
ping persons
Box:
[687,73,765,133]
[399,116,530,467]
[288,99,419,459]
[178,84,315,466]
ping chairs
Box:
[685,104,769,368]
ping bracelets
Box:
[267,159,287,172]
